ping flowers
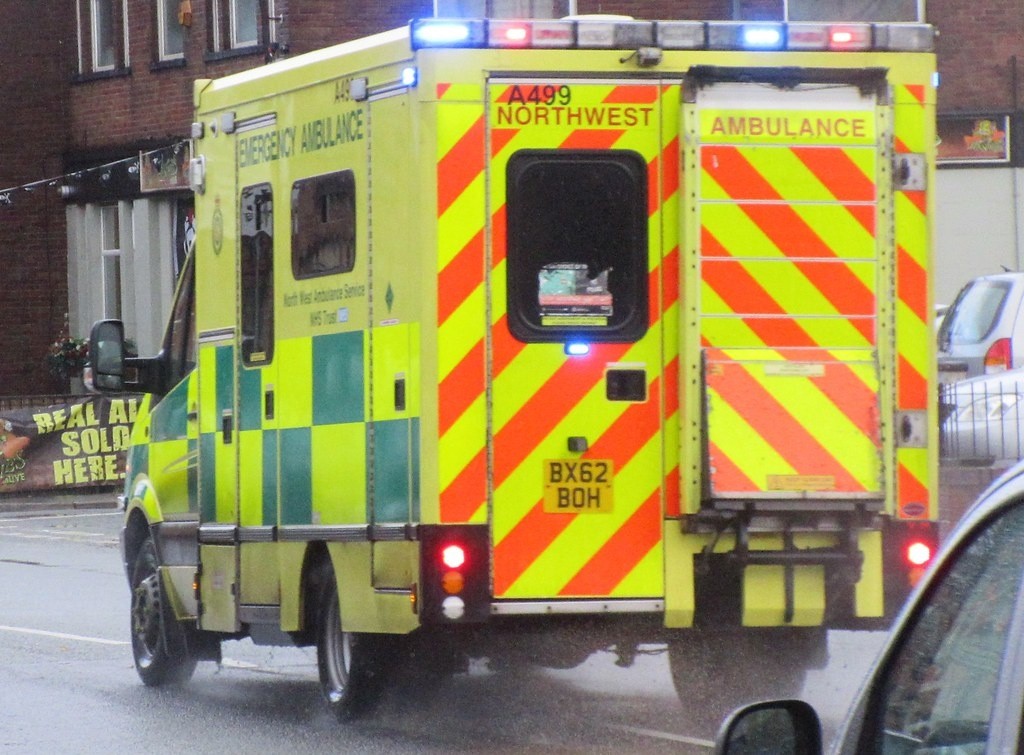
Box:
[46,337,90,388]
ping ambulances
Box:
[118,10,941,730]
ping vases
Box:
[70,377,88,394]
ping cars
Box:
[939,368,1024,460]
[713,460,1024,755]
[937,264,1024,384]
